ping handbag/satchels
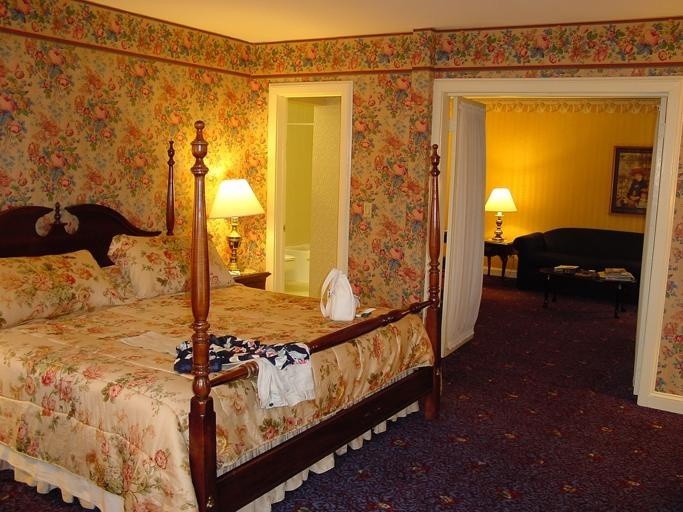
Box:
[319,268,355,322]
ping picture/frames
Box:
[610,146,653,214]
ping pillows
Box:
[0,229,236,329]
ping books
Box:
[554,265,635,281]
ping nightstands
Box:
[233,272,272,290]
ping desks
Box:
[484,241,513,280]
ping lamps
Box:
[484,187,519,243]
[207,179,266,277]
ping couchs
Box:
[513,227,644,304]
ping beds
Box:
[0,120,442,511]
